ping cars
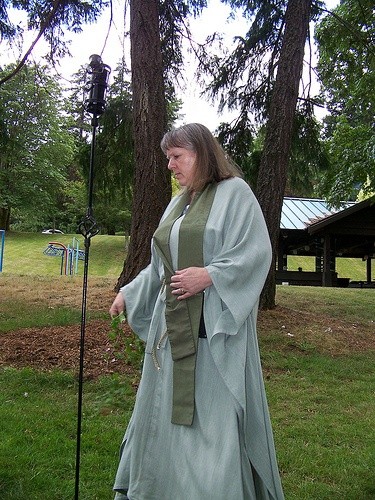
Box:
[42,229,64,235]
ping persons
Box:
[108,123,287,500]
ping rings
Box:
[180,288,184,295]
